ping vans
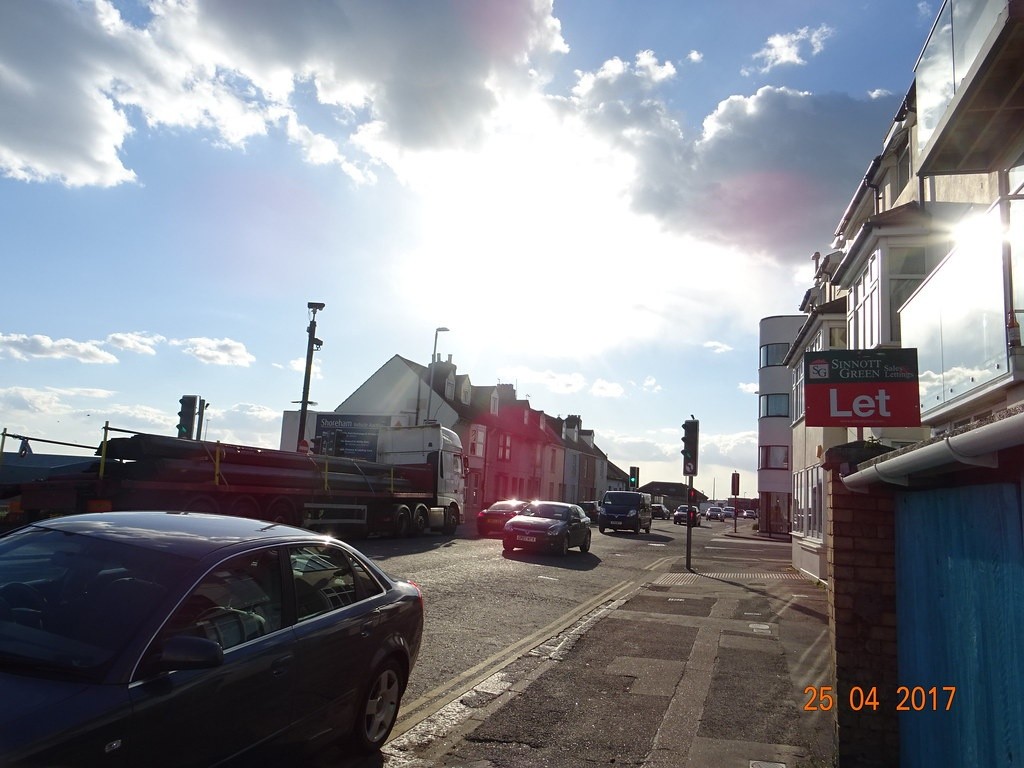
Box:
[597,491,652,535]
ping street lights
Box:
[426,327,449,419]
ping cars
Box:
[502,500,592,558]
[706,507,725,522]
[723,507,736,518]
[476,500,530,535]
[651,504,670,520]
[743,510,756,520]
[0,510,425,768]
[673,505,701,527]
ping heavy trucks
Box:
[0,422,470,538]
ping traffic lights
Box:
[689,511,697,525]
[681,420,698,476]
[687,488,697,502]
[629,466,637,488]
[175,395,197,440]
